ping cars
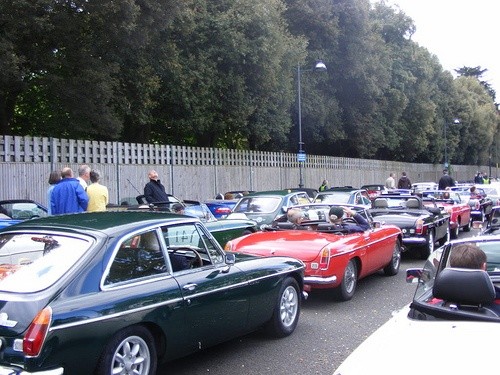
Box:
[332,235,500,375]
[223,203,404,302]
[106,182,500,262]
[0,209,309,375]
[0,198,49,230]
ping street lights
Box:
[445,118,460,169]
[296,61,328,187]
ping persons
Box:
[50,167,88,215]
[87,170,109,211]
[144,170,170,208]
[439,169,455,190]
[141,231,187,273]
[76,165,90,189]
[385,173,396,189]
[318,180,328,192]
[450,244,500,299]
[471,187,477,192]
[173,203,185,214]
[329,207,370,233]
[287,208,306,229]
[475,172,487,184]
[47,169,62,215]
[216,193,233,200]
[399,172,411,189]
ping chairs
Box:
[215,192,224,200]
[373,199,389,209]
[121,201,128,206]
[17,209,33,219]
[138,231,167,275]
[235,193,244,199]
[432,267,499,318]
[250,203,262,212]
[406,198,420,209]
[317,223,336,230]
[277,222,296,230]
